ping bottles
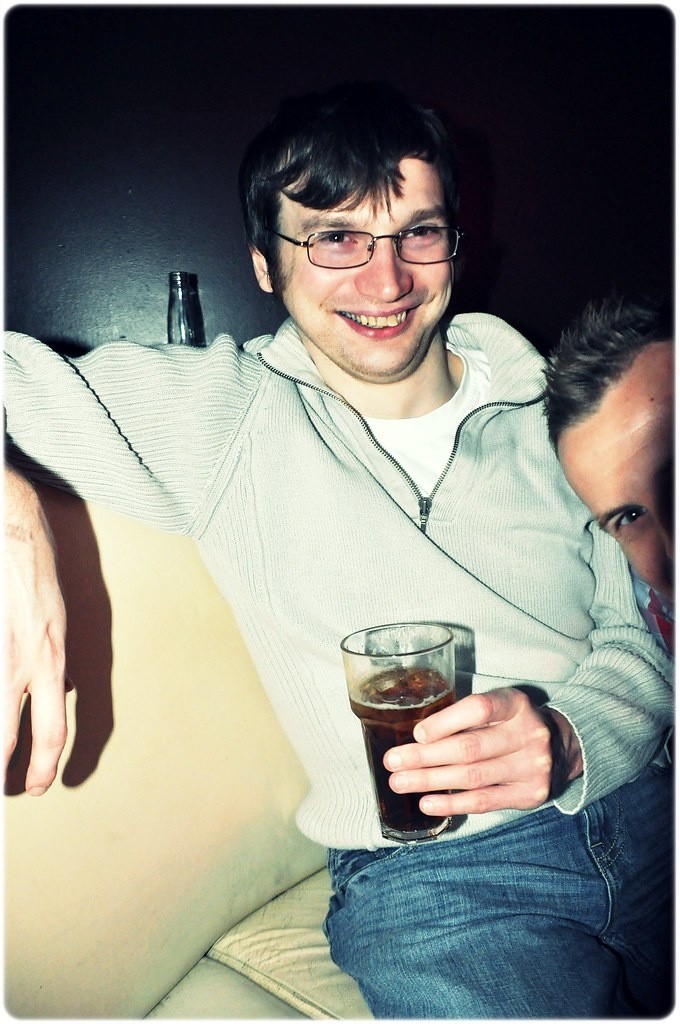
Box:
[168,272,194,348]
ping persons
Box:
[539,293,674,603]
[5,81,674,1018]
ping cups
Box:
[343,620,456,842]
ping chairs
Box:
[3,485,374,1019]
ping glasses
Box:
[272,225,466,269]
